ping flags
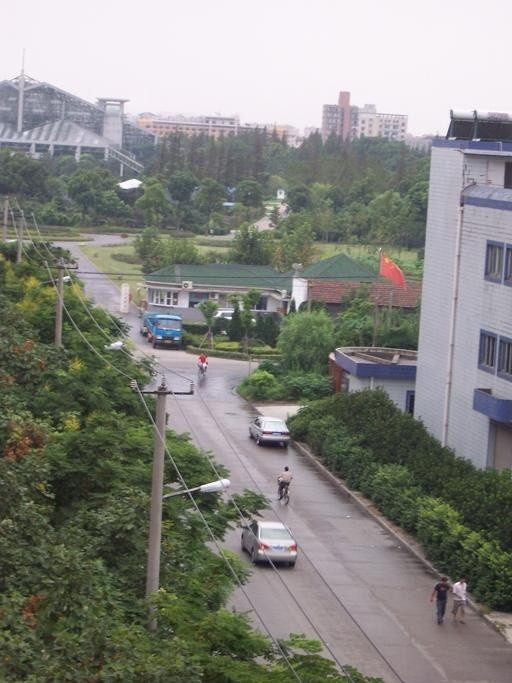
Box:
[378,250,408,291]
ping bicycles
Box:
[198,367,206,380]
[277,484,290,506]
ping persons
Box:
[277,465,293,501]
[449,574,467,625]
[197,351,208,376]
[430,575,452,625]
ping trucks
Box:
[139,312,182,350]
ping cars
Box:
[249,417,291,448]
[241,521,297,567]
[213,310,257,323]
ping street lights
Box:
[53,340,123,364]
[41,275,71,290]
[145,478,231,630]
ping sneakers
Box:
[279,496,283,500]
